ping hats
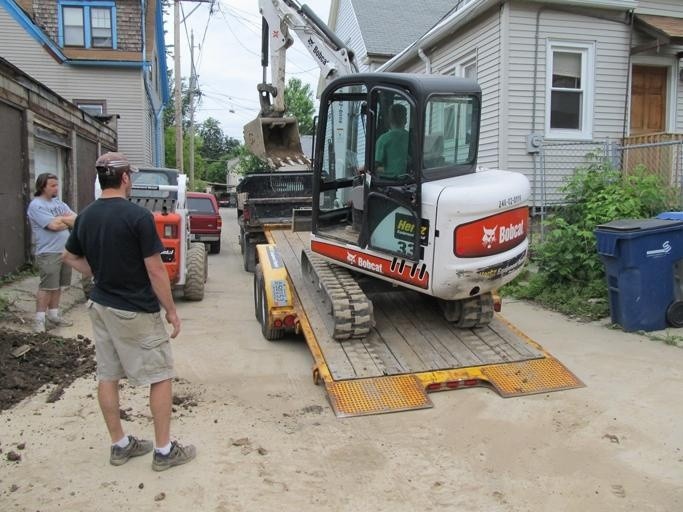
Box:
[96,150,139,176]
[33,173,58,196]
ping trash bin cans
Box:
[592,212,683,333]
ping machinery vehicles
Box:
[128,167,208,301]
[243,1,531,340]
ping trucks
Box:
[236,171,586,418]
[215,192,230,206]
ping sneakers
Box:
[151,442,197,472]
[33,319,48,335]
[108,434,152,467]
[47,316,74,327]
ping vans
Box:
[186,192,222,254]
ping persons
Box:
[62,152,196,472]
[25,173,77,333]
[345,103,409,234]
